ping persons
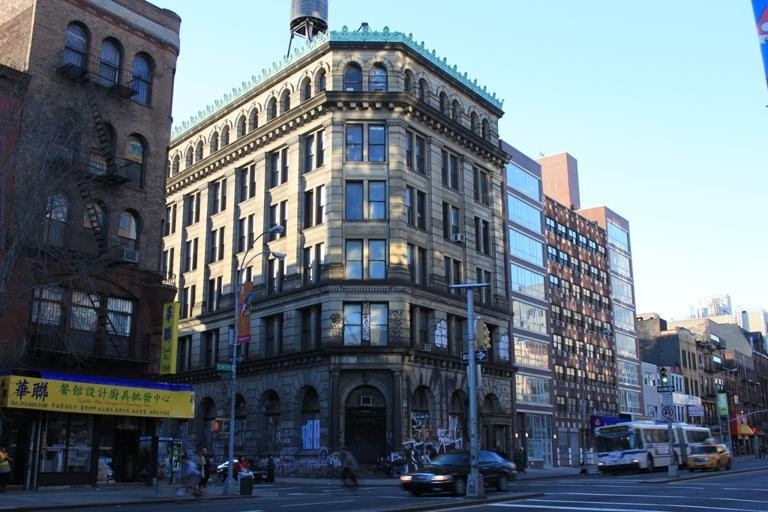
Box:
[174,448,211,492]
[339,447,359,488]
[517,447,527,473]
[266,459,276,482]
[740,445,744,458]
[0,446,13,492]
[235,455,250,487]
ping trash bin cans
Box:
[238,472,254,495]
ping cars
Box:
[686,436,734,475]
[215,457,269,483]
[397,448,520,497]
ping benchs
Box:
[35,472,97,490]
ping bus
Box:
[592,421,713,473]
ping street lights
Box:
[223,221,291,496]
[713,367,739,443]
[447,281,498,502]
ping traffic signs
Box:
[655,385,677,393]
[215,363,233,372]
[460,349,490,363]
[228,354,246,364]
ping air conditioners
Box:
[360,396,372,406]
[119,247,139,263]
[453,233,465,243]
[419,343,432,353]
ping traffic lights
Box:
[660,366,669,386]
[211,418,224,434]
